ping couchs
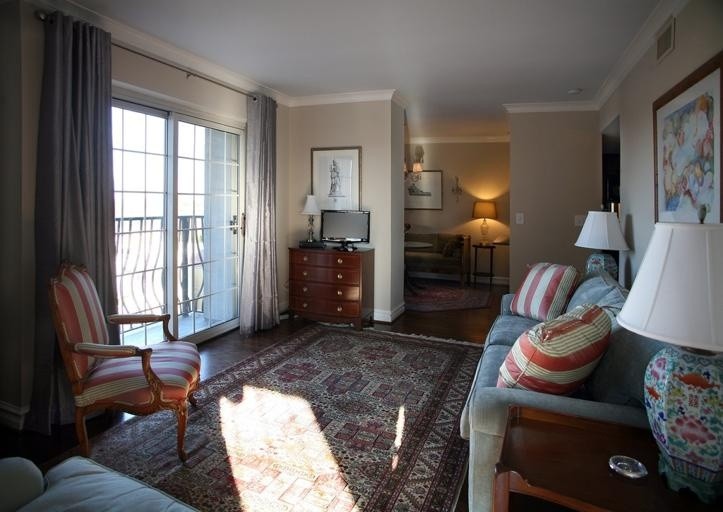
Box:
[405,231,471,286]
[0,453,200,512]
[460,273,674,512]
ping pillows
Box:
[439,234,463,257]
[510,262,580,323]
[498,302,611,392]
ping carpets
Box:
[64,322,486,512]
[402,283,495,313]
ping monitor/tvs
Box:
[319,209,371,251]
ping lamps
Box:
[616,220,722,505]
[574,210,630,278]
[472,201,497,245]
[411,162,423,173]
[296,195,324,249]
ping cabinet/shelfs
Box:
[287,246,374,332]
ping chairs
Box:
[48,258,202,463]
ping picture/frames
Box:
[311,146,361,212]
[651,50,723,226]
[402,170,443,210]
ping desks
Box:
[489,403,723,512]
[403,241,434,295]
[470,244,496,290]
